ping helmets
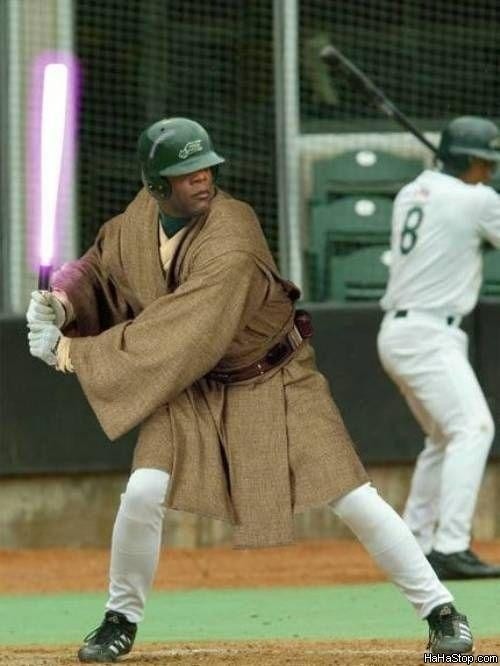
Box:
[138,118,226,200]
[437,116,500,162]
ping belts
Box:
[206,325,302,384]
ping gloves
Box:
[26,288,77,374]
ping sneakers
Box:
[424,601,474,657]
[77,609,137,662]
[425,550,500,578]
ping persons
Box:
[25,116,474,663]
[376,115,500,580]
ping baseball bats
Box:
[37,63,68,290]
[318,45,442,160]
